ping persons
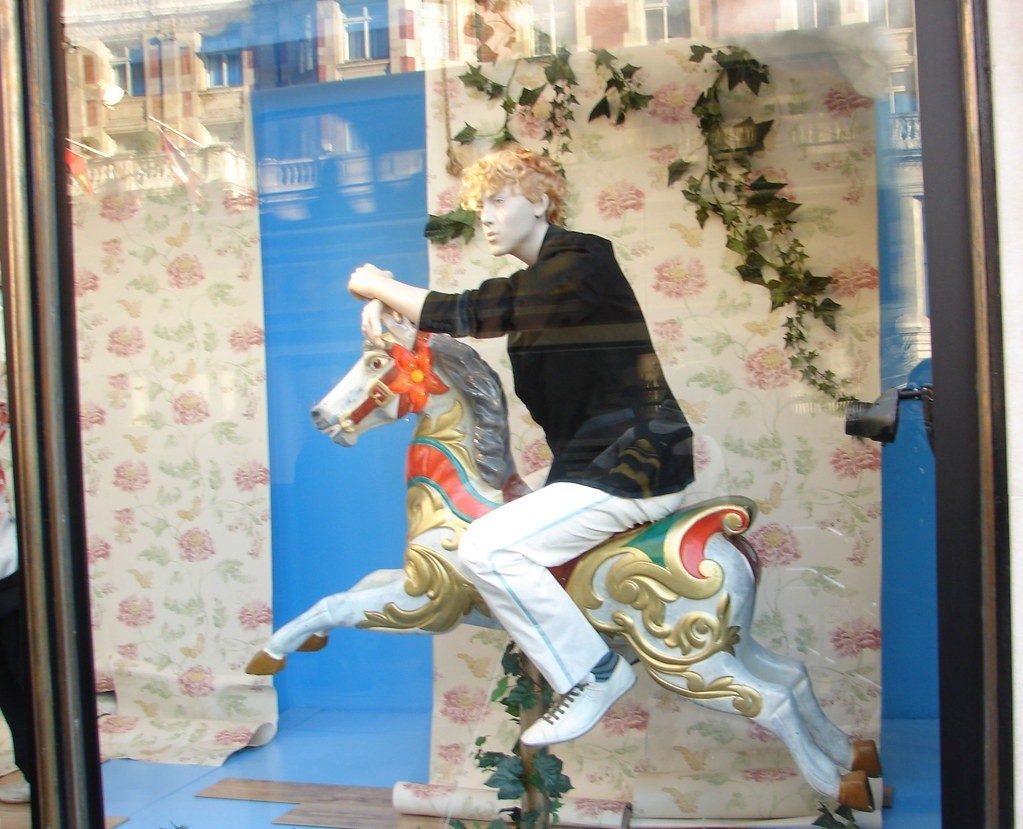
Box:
[346,150,697,748]
[0,370,39,829]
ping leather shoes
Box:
[520,653,638,746]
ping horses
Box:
[241,270,885,815]
